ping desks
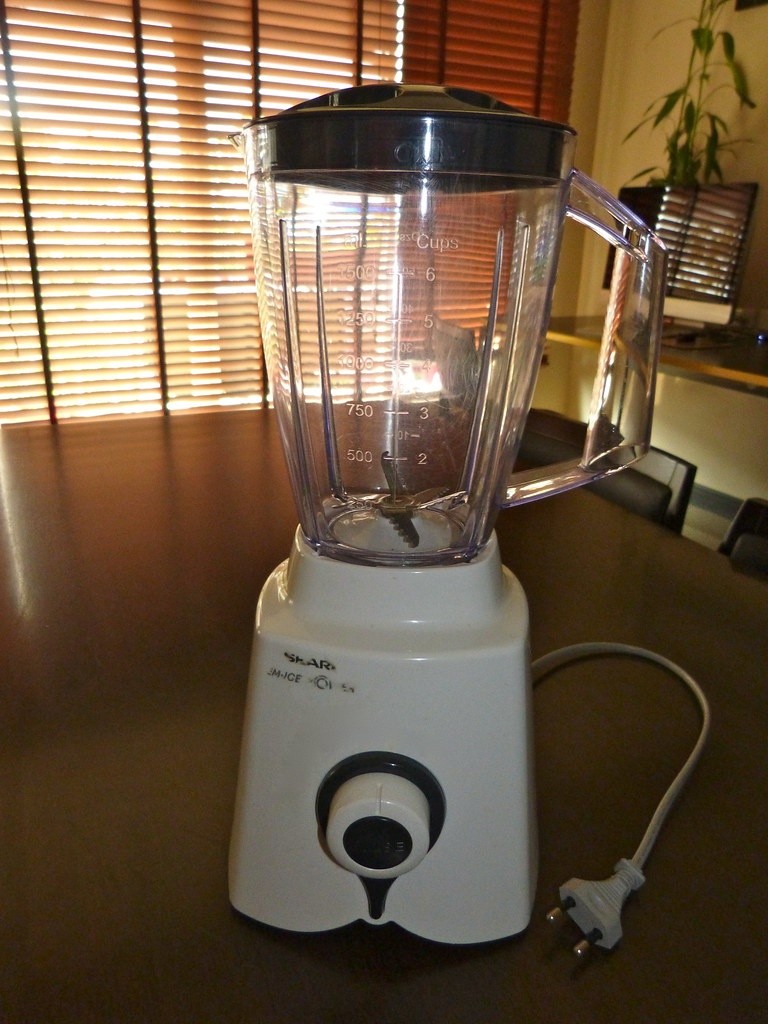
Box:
[544,316,768,398]
[0,401,768,1024]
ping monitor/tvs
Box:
[600,178,758,334]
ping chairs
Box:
[511,406,697,536]
[716,496,768,582]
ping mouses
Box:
[675,329,697,342]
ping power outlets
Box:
[730,308,758,328]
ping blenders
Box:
[226,82,669,952]
